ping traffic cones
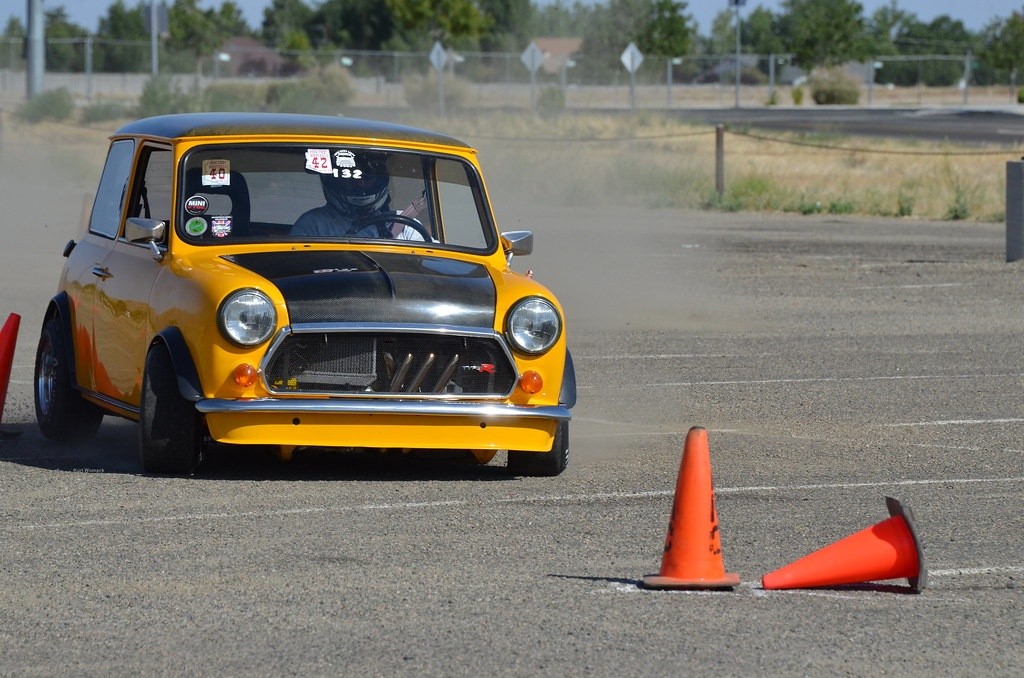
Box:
[760,495,928,595]
[0,312,21,423]
[641,426,741,589]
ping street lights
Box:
[869,61,884,104]
[666,57,682,109]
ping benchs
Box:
[154,220,295,244]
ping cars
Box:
[32,111,576,477]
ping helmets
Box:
[320,148,392,216]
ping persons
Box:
[290,150,439,244]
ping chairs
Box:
[182,167,251,243]
